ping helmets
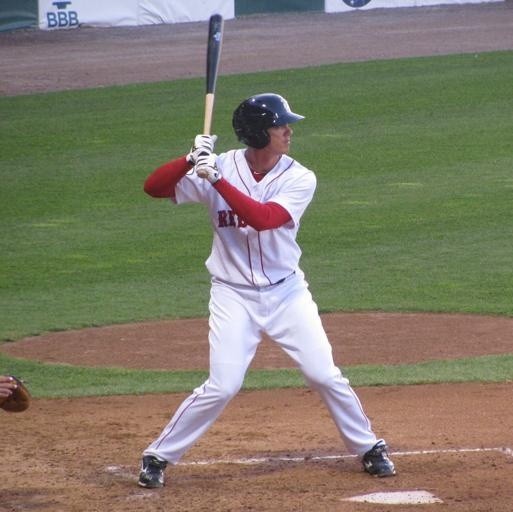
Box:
[228,92,306,146]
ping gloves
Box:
[185,133,226,185]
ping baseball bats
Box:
[198,15,225,177]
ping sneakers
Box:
[362,439,396,477]
[136,453,167,488]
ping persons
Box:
[0,374,18,398]
[137,92,398,488]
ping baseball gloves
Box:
[0,377,31,413]
